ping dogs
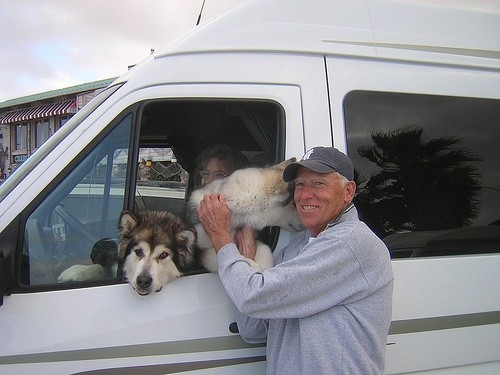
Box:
[53,210,204,301]
[181,153,306,284]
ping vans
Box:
[1,1,500,375]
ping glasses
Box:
[289,178,333,191]
[199,168,228,182]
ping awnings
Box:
[1,99,76,125]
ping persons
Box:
[197,147,394,375]
[193,145,250,189]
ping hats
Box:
[281,146,357,185]
